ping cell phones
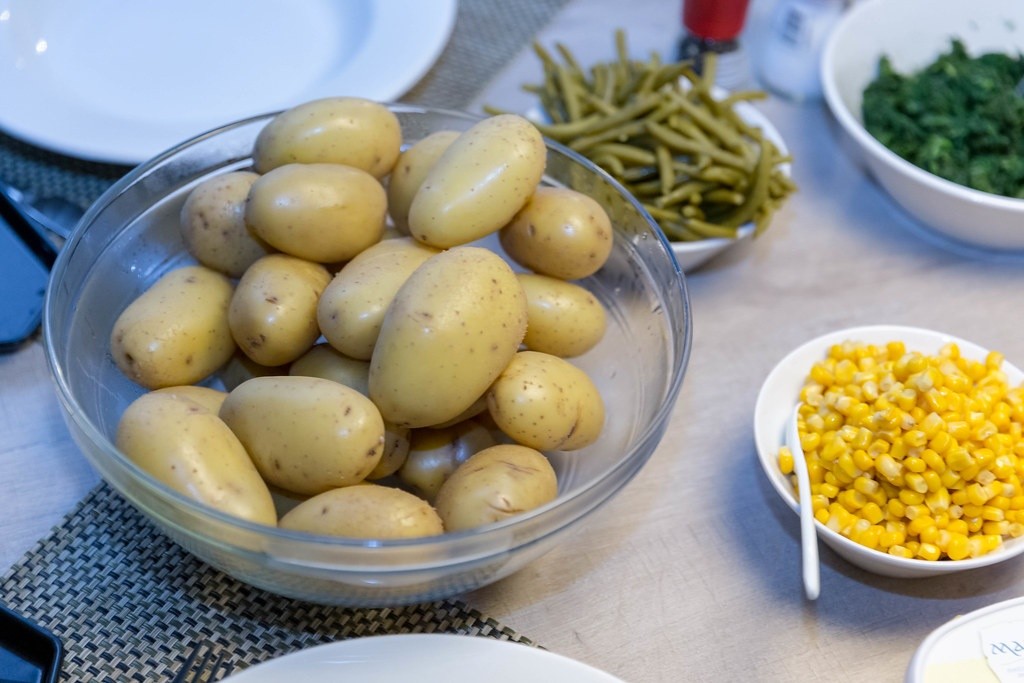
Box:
[1,601,62,683]
[1,192,59,352]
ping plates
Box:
[217,633,622,682]
[0,0,462,166]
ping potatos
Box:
[105,95,613,606]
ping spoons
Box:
[786,402,821,604]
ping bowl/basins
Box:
[40,101,693,611]
[528,78,794,280]
[817,1,1024,254]
[752,326,1023,577]
